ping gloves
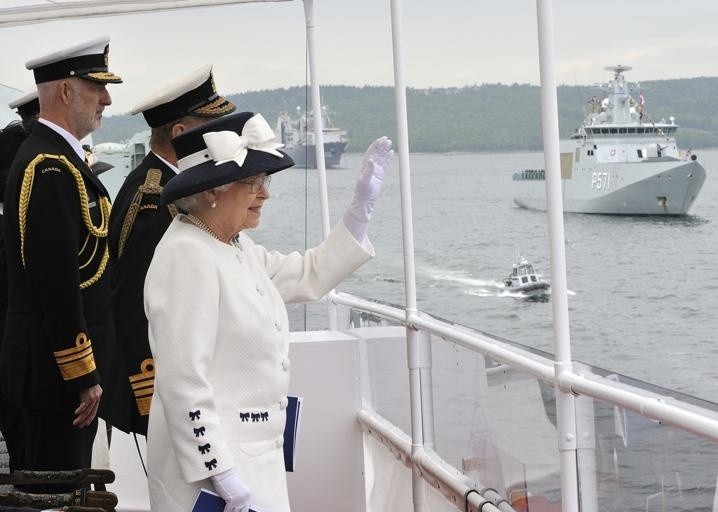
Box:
[347,133,396,222]
[210,467,253,512]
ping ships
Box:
[512,63,707,217]
[272,96,349,168]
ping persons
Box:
[12,90,45,133]
[0,121,25,204]
[143,111,394,511]
[0,35,112,510]
[100,69,232,435]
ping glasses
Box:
[235,175,272,194]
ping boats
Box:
[120,132,153,186]
[503,255,550,294]
[1,6,718,512]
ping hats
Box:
[127,60,240,122]
[22,32,124,101]
[6,89,45,120]
[155,110,297,210]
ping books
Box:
[191,488,260,511]
[283,395,304,473]
